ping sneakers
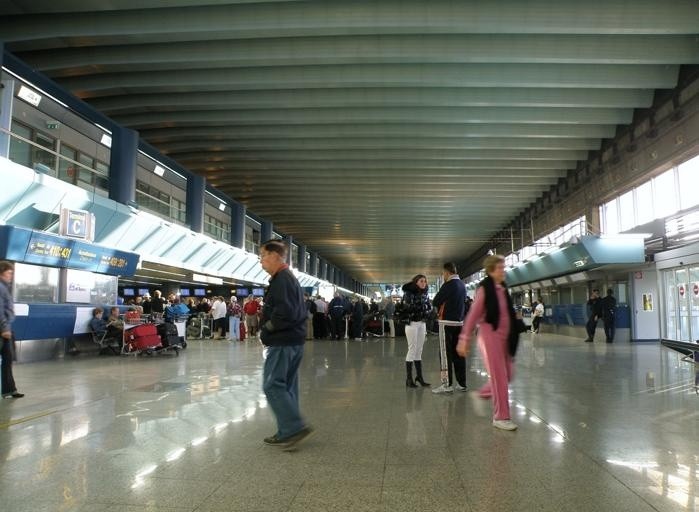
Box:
[281,425,316,451]
[262,429,289,446]
[606,338,613,343]
[454,383,468,393]
[470,390,489,418]
[585,337,593,342]
[491,418,518,433]
[431,384,455,394]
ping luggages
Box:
[123,321,186,351]
[187,316,211,339]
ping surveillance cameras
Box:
[487,250,494,256]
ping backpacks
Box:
[604,299,616,324]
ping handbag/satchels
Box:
[389,302,413,325]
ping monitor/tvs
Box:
[192,288,206,297]
[252,287,265,298]
[179,288,190,297]
[235,288,249,297]
[138,288,149,296]
[123,288,135,297]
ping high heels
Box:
[415,376,431,387]
[1,392,25,399]
[405,378,418,389]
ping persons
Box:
[401,274,433,388]
[601,289,616,344]
[259,241,317,452]
[584,289,602,342]
[0,260,24,399]
[89,290,265,353]
[532,298,544,334]
[302,291,402,341]
[529,297,544,333]
[456,255,523,431]
[432,262,468,394]
[464,296,473,315]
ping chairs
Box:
[85,318,119,356]
